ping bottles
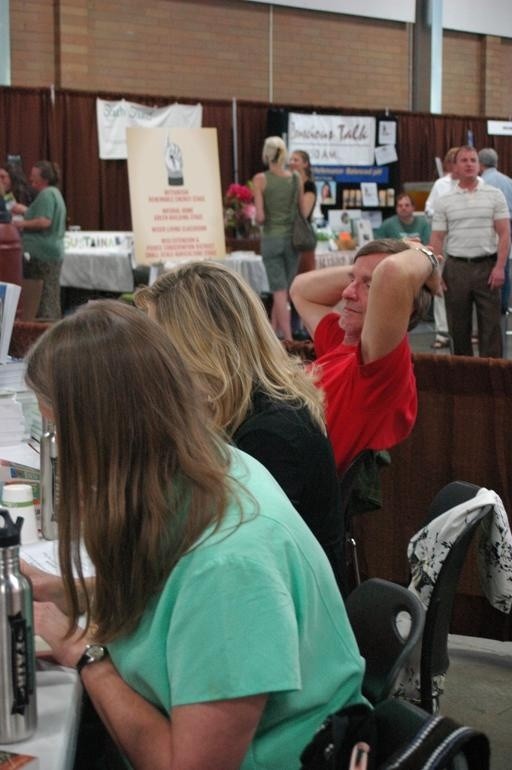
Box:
[40,429,60,540]
[0,509,37,744]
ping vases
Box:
[227,237,260,253]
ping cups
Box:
[3,484,38,545]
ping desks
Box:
[55,227,373,318]
[0,353,91,770]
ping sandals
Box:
[430,339,449,348]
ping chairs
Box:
[416,482,490,714]
[337,579,425,705]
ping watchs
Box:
[75,643,108,672]
[416,244,438,278]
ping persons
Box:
[424,147,510,358]
[478,149,512,314]
[12,161,67,322]
[19,300,374,770]
[288,240,445,478]
[430,148,482,349]
[290,150,319,339]
[376,194,430,247]
[254,136,307,342]
[134,265,348,595]
[0,169,35,221]
[321,182,334,204]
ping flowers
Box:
[225,183,258,239]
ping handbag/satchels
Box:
[292,210,317,252]
[294,700,493,770]
